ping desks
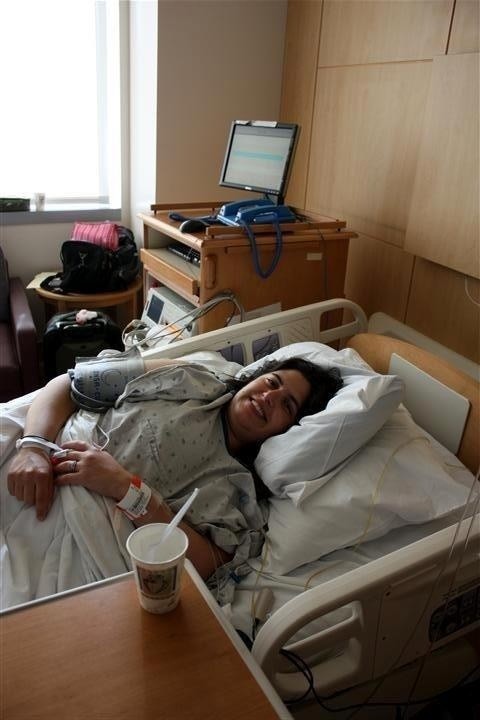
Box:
[2,557,303,719]
[36,269,140,354]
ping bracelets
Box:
[116,479,165,522]
[15,435,63,453]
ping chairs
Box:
[1,241,39,399]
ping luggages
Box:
[41,310,124,384]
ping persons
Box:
[8,359,344,581]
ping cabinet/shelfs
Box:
[135,208,355,359]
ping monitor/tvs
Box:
[220,117,299,204]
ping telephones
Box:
[217,199,297,227]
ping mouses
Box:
[180,216,208,236]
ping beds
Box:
[1,298,479,699]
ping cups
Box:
[126,524,189,616]
[33,192,45,211]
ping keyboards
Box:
[163,240,202,270]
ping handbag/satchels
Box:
[60,226,140,295]
